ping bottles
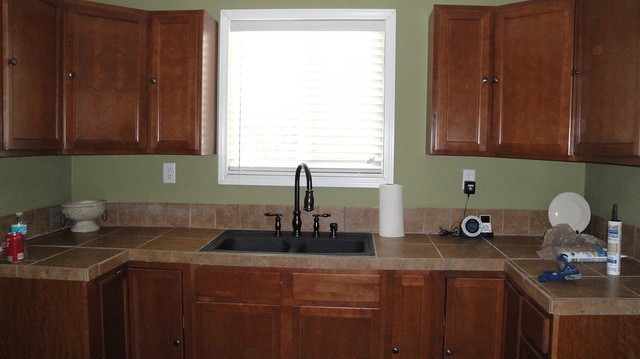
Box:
[12,212,28,257]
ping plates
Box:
[548,192,592,234]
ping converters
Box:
[464,180,476,194]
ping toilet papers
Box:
[379,183,405,238]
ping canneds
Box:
[5,231,24,264]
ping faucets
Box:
[292,163,314,235]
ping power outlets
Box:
[463,170,475,191]
[163,163,175,183]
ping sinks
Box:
[201,229,376,257]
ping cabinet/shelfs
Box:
[380,269,447,359]
[149,9,216,155]
[0,263,129,359]
[185,265,380,359]
[576,0,640,166]
[62,0,149,154]
[425,1,576,162]
[505,275,640,359]
[447,270,506,359]
[0,0,63,158]
[129,261,185,359]
